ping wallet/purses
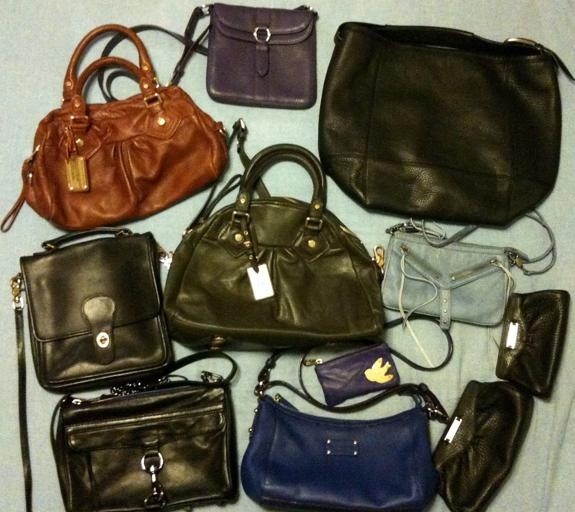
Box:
[315,345,398,409]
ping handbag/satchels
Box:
[53,371,241,509]
[22,25,229,232]
[20,227,174,394]
[241,392,436,510]
[494,288,569,399]
[207,3,317,110]
[318,21,570,229]
[429,380,534,512]
[162,143,385,349]
[379,225,528,327]
